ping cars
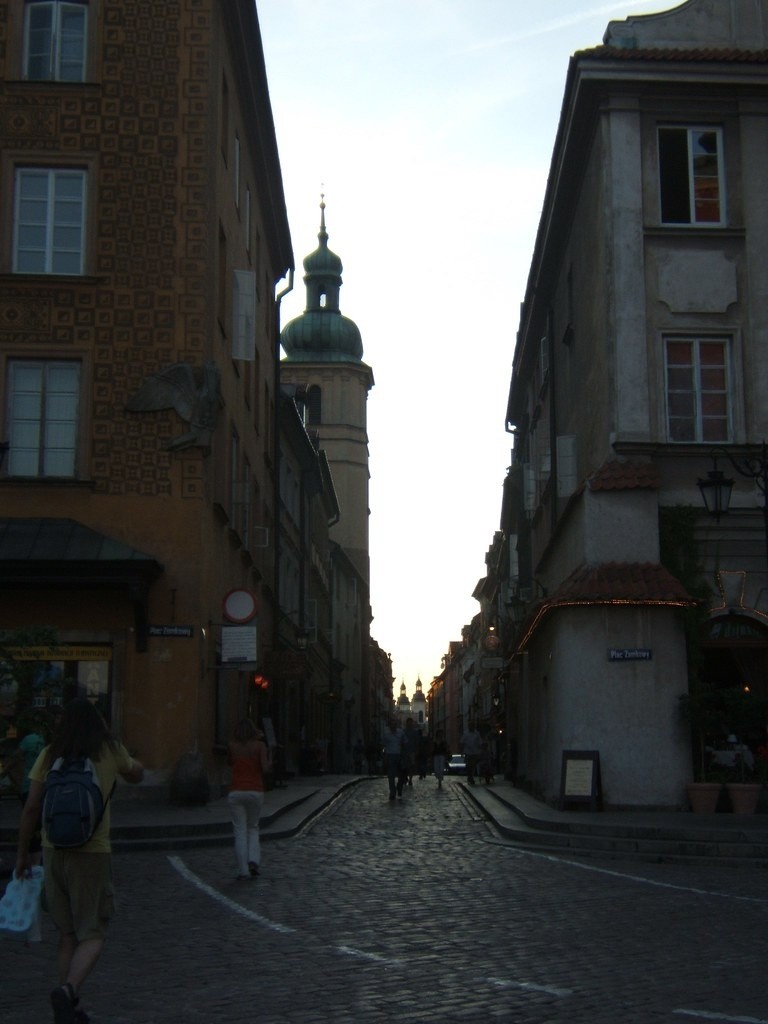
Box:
[448,754,468,775]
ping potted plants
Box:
[681,680,768,816]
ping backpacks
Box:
[39,741,117,849]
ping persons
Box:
[353,718,448,802]
[461,725,492,785]
[758,734,768,759]
[0,697,144,1024]
[227,718,273,881]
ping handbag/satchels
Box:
[258,749,276,793]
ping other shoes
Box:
[409,781,412,785]
[249,863,261,876]
[389,793,395,800]
[397,788,402,796]
[236,875,247,881]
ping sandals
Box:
[50,983,91,1024]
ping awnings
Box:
[1,516,165,595]
